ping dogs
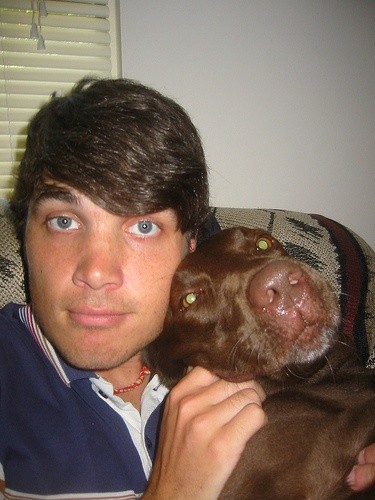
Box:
[146,213,375,500]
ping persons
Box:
[0,75,375,500]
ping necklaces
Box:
[114,366,151,396]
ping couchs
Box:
[0,207,375,376]
